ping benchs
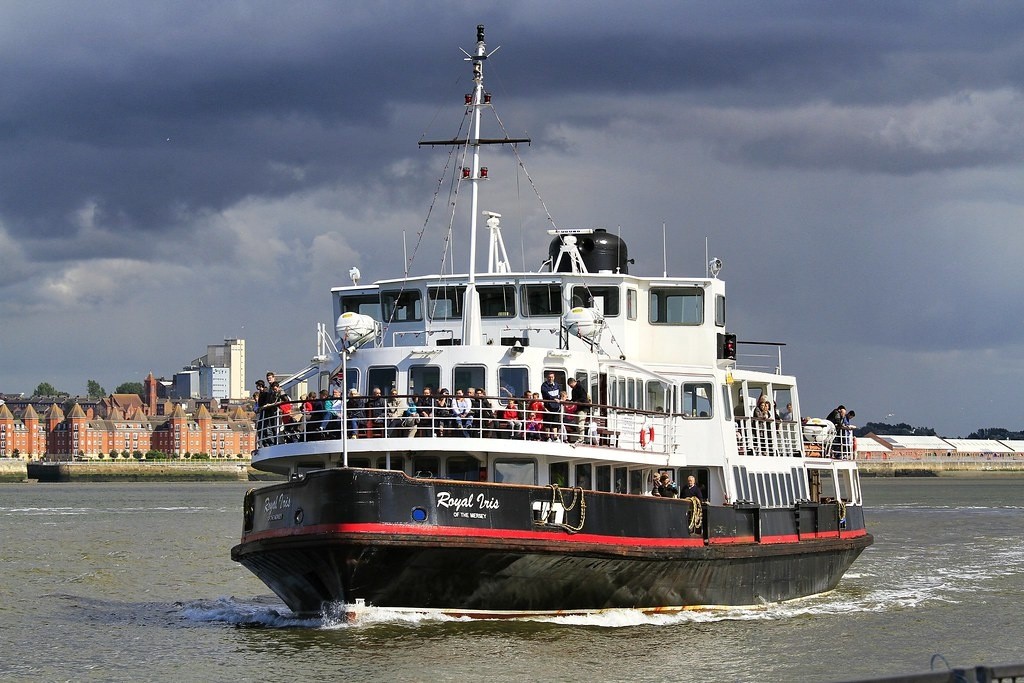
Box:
[345,406,613,447]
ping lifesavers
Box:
[640,423,655,449]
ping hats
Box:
[652,473,660,479]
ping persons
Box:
[680,476,703,501]
[252,372,297,447]
[651,471,678,498]
[734,395,792,456]
[826,405,855,459]
[504,372,599,446]
[283,388,500,442]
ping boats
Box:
[230,24,873,627]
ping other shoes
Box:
[349,435,357,439]
[546,437,553,442]
[553,437,560,442]
[316,427,324,431]
[574,438,584,445]
[439,431,444,436]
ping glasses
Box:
[457,393,461,394]
[476,393,481,394]
[376,393,381,394]
[467,392,472,393]
